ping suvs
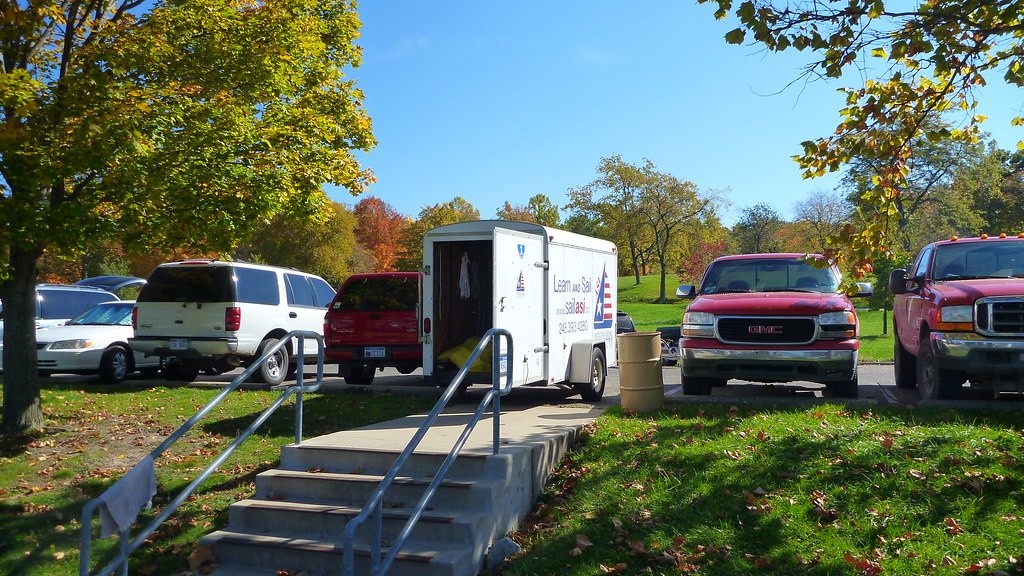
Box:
[674,252,875,399]
[127,257,339,384]
[0,275,149,379]
[616,311,637,333]
[883,233,1024,401]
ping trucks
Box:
[418,220,619,407]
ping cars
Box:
[323,273,422,384]
[656,325,683,366]
[34,299,162,381]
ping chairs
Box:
[941,264,964,279]
[796,277,819,289]
[728,281,749,292]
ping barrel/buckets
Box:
[618,331,666,413]
